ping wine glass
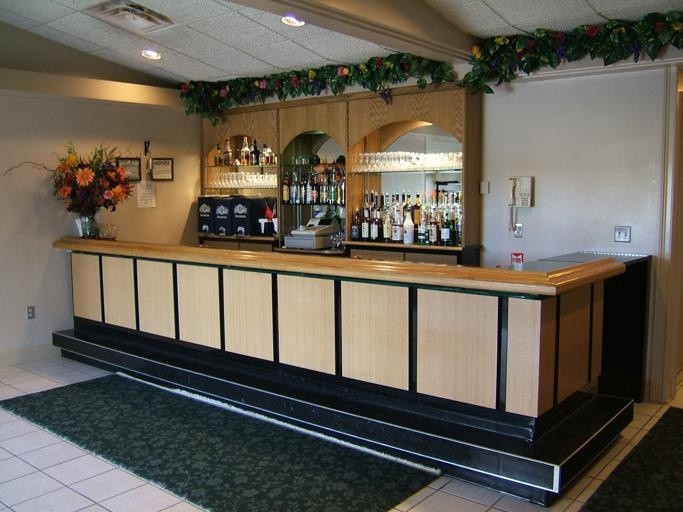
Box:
[211,171,277,188]
[330,234,338,251]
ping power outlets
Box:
[613,226,631,242]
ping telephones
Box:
[508,176,533,208]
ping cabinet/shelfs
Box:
[200,109,278,253]
[274,101,349,253]
[342,88,483,263]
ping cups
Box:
[352,151,462,172]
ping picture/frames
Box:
[150,158,174,182]
[116,157,142,183]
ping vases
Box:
[81,215,102,238]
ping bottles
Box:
[351,191,462,246]
[282,165,345,205]
[213,137,277,166]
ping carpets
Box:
[577,405,683,512]
[0,372,439,512]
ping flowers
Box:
[179,51,456,116]
[462,9,683,99]
[2,141,138,215]
